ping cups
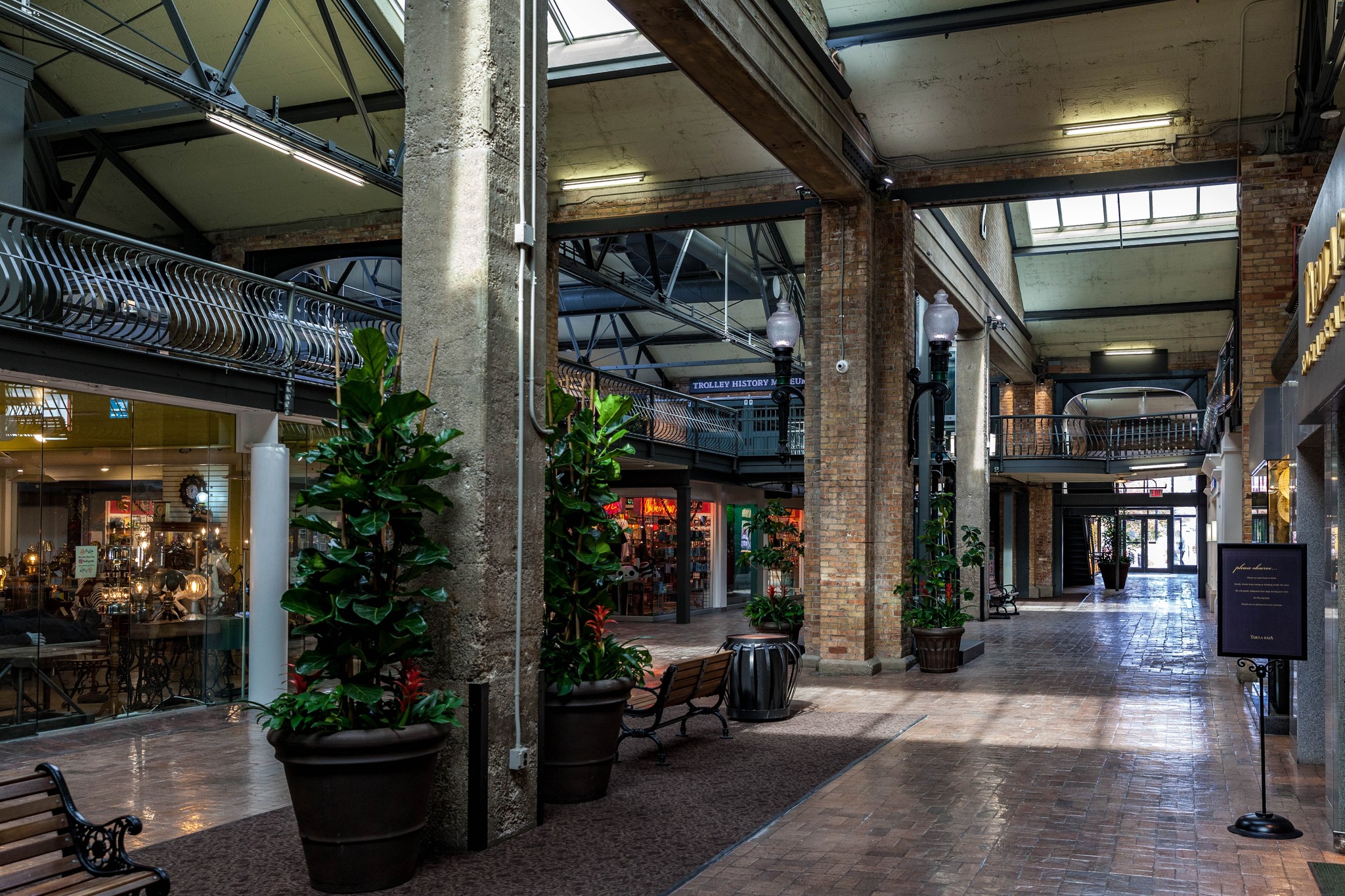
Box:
[986,594,990,600]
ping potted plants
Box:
[891,489,989,674]
[537,386,658,804]
[734,495,807,666]
[1087,509,1167,589]
[232,324,472,894]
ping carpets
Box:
[1307,861,1345,896]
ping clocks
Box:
[152,501,172,521]
[178,472,209,509]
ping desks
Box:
[93,611,135,718]
[0,642,108,742]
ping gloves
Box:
[27,632,46,645]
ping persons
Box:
[1174,530,1186,565]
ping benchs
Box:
[614,645,742,762]
[988,577,1020,619]
[0,761,172,896]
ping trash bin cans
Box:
[713,633,803,722]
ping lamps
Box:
[204,112,366,189]
[1061,114,1177,139]
[23,542,42,576]
[136,526,151,577]
[53,543,75,578]
[17,468,24,473]
[100,465,110,471]
[558,173,648,191]
[148,568,188,622]
[1103,349,1155,356]
[181,568,210,622]
[906,286,959,467]
[1138,390,1147,419]
[131,576,151,625]
[769,298,805,465]
[34,540,56,576]
